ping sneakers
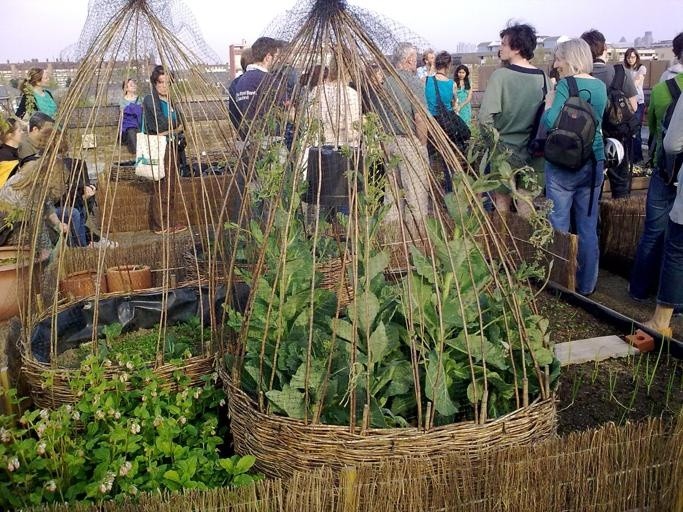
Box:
[89,237,119,249]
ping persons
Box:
[477,23,683,338]
[0,65,185,260]
[230,37,473,223]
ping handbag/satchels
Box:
[437,108,471,144]
[527,91,555,159]
[136,132,167,182]
[0,219,15,246]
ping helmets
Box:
[603,138,624,168]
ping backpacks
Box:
[543,76,600,174]
[657,79,683,189]
[588,65,642,140]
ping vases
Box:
[60,269,107,302]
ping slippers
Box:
[153,224,185,234]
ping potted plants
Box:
[107,263,152,292]
[0,201,50,322]
